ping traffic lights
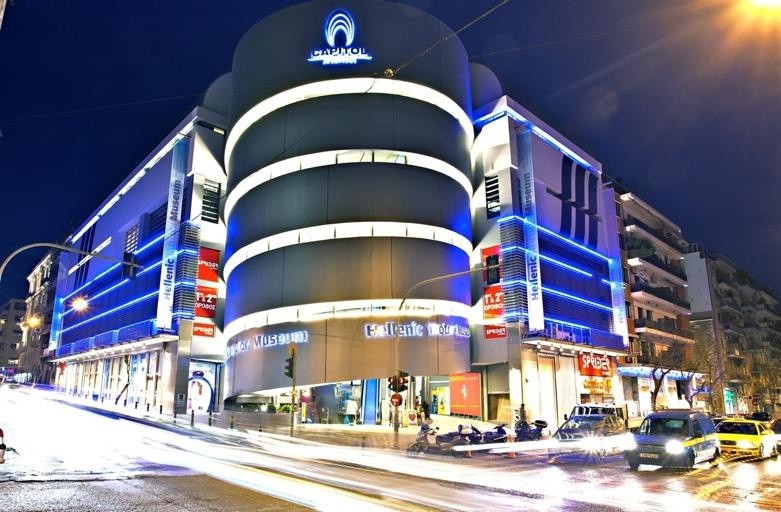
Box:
[388,375,408,392]
[284,358,293,377]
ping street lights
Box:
[26,319,36,382]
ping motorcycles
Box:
[406,416,548,455]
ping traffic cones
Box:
[465,444,472,458]
[507,434,516,458]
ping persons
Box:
[343,395,358,424]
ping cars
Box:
[548,402,781,472]
